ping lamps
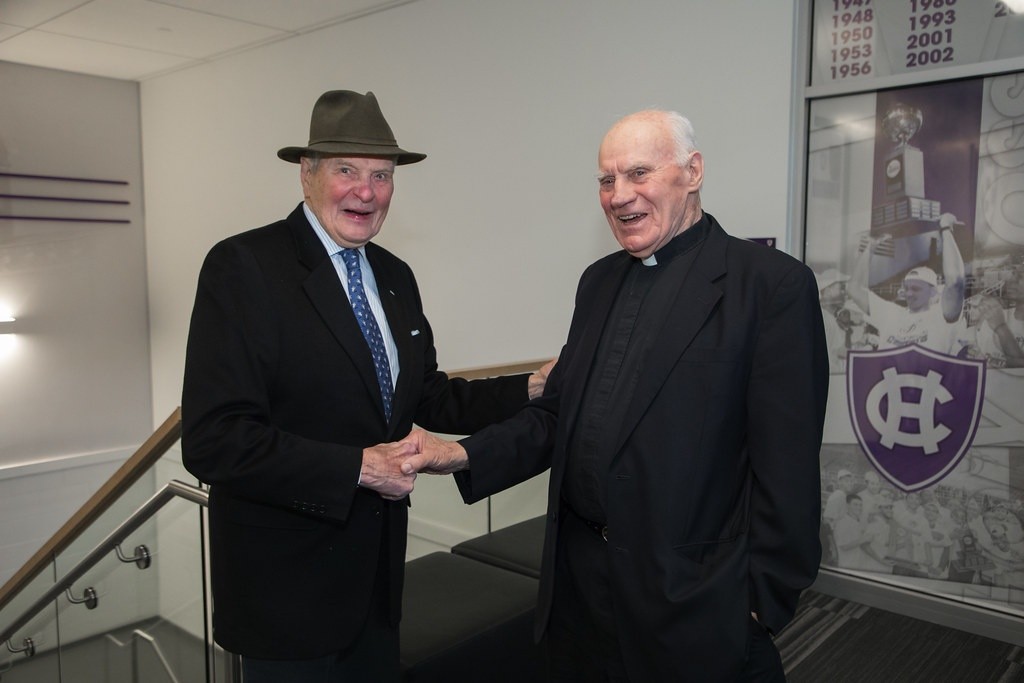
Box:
[0,317,18,335]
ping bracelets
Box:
[994,323,1006,332]
[939,227,953,237]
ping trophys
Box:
[870,102,965,239]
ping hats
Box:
[278,90,428,166]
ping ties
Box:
[337,249,396,424]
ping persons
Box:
[180,90,559,683]
[825,469,1023,583]
[403,109,829,683]
[817,212,1024,374]
